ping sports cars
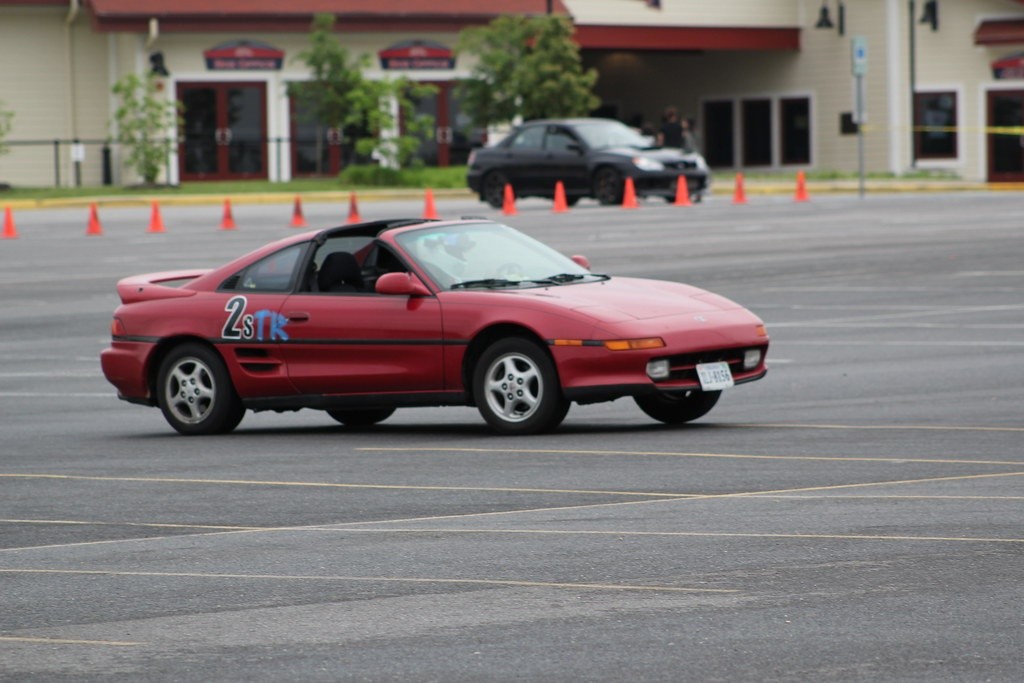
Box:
[99,216,771,434]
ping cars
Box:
[464,118,711,206]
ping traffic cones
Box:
[733,173,747,204]
[500,184,519,214]
[1,206,18,239]
[83,202,104,235]
[346,192,364,224]
[553,180,569,214]
[220,200,237,230]
[669,175,694,207]
[621,176,638,209]
[423,190,439,219]
[794,171,809,201]
[146,202,166,233]
[290,199,308,228]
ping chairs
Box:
[316,252,364,295]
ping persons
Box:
[640,104,697,152]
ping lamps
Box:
[814,0,846,35]
[919,0,940,31]
[147,51,169,78]
